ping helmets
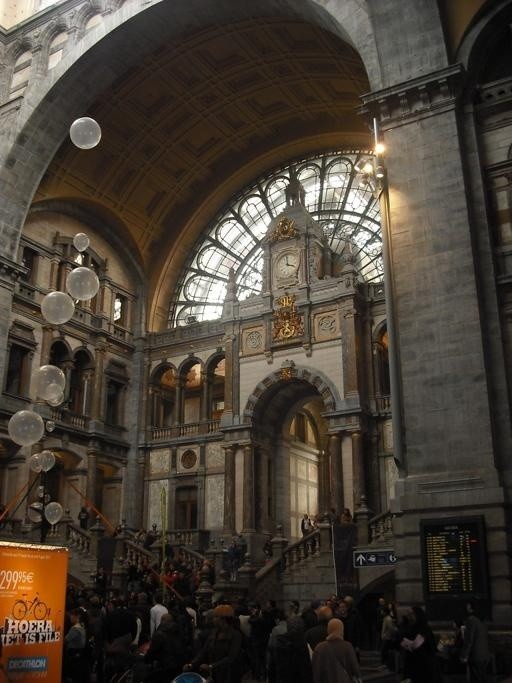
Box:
[211,605,234,616]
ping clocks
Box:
[275,250,300,278]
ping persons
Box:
[40,493,52,543]
[374,595,437,683]
[458,597,499,682]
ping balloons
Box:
[41,291,75,325]
[29,450,55,474]
[72,232,89,252]
[32,364,66,406]
[70,116,102,150]
[26,485,63,524]
[8,410,44,448]
[46,421,56,433]
[65,267,100,301]
